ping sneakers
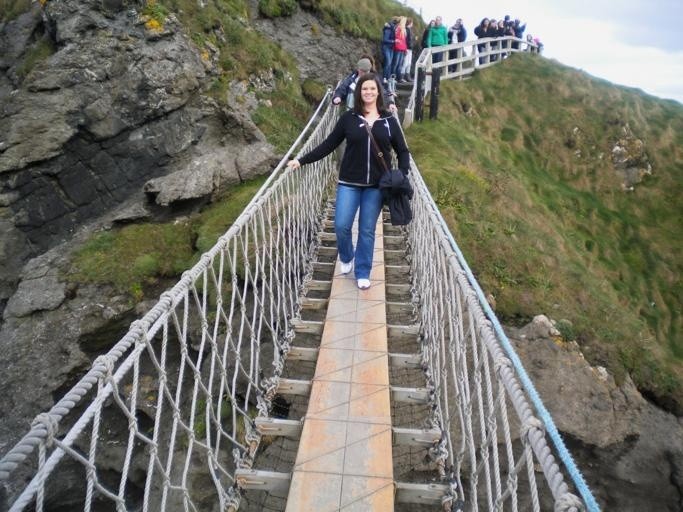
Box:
[357,279,371,289]
[340,259,353,274]
[383,78,387,83]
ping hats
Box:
[356,58,372,71]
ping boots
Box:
[399,74,406,83]
[405,74,413,82]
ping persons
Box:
[330,57,398,119]
[361,54,398,102]
[281,73,411,293]
[379,14,542,86]
